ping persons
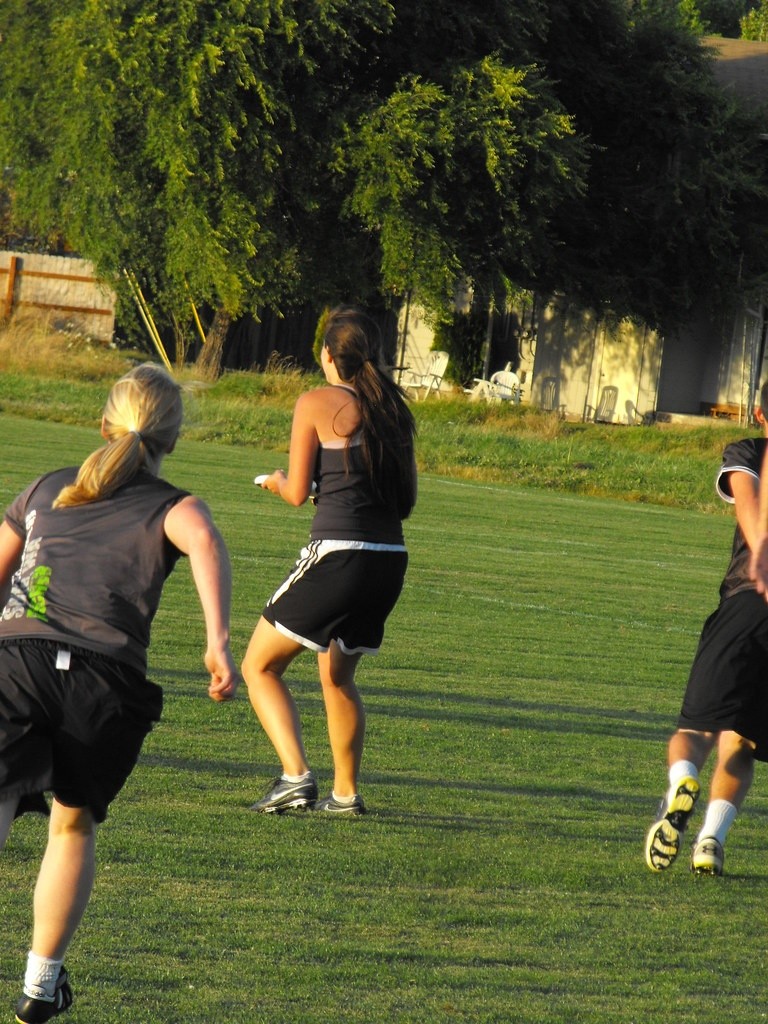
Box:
[241,308,417,815]
[644,381,768,875]
[1,364,240,1024]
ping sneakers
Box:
[691,837,724,877]
[645,777,702,873]
[13,963,74,1024]
[247,777,319,815]
[313,795,366,814]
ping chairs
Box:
[490,370,520,405]
[401,351,450,402]
